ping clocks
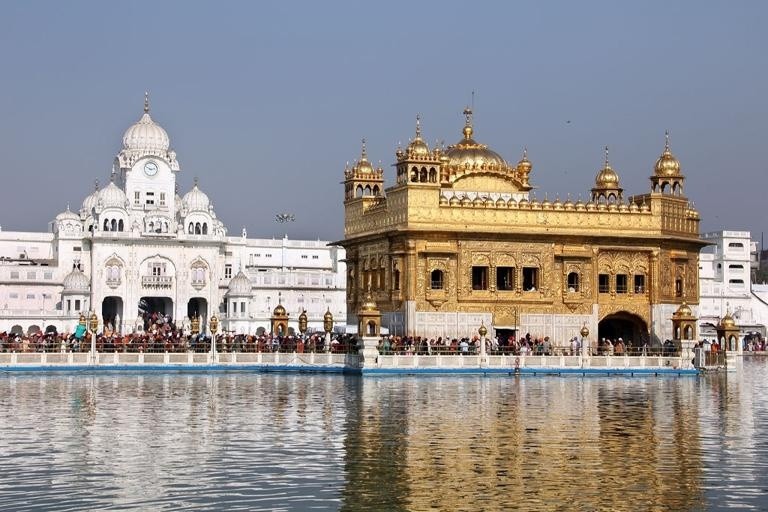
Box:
[143,162,158,177]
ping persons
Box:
[674,265,683,297]
[527,284,536,291]
[752,279,768,285]
[568,285,576,293]
[743,331,768,352]
